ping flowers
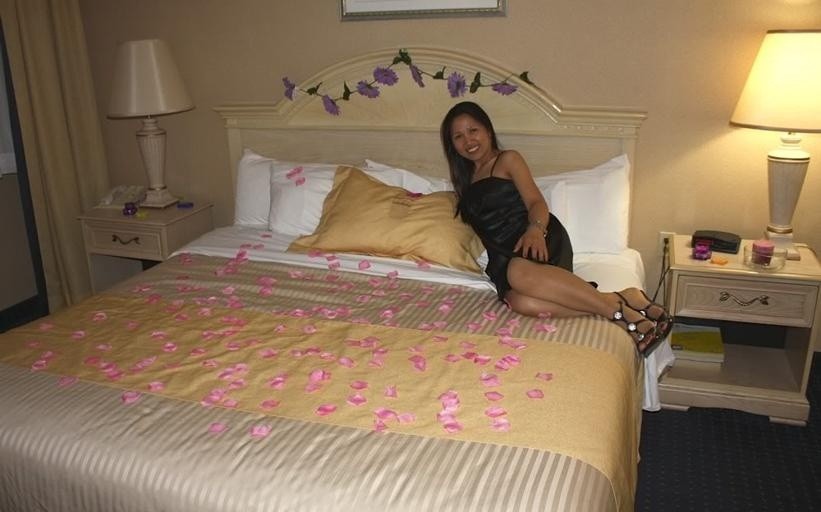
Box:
[281,47,536,116]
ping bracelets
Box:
[534,219,548,237]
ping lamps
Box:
[104,37,197,208]
[730,29,821,260]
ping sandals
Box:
[609,301,663,358]
[614,290,673,341]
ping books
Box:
[671,352,724,364]
[668,324,725,357]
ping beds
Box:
[1,43,651,511]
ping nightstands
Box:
[73,201,215,296]
[654,233,821,430]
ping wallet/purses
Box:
[691,231,741,253]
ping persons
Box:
[440,101,673,357]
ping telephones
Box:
[99,184,147,210]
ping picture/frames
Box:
[338,0,509,21]
